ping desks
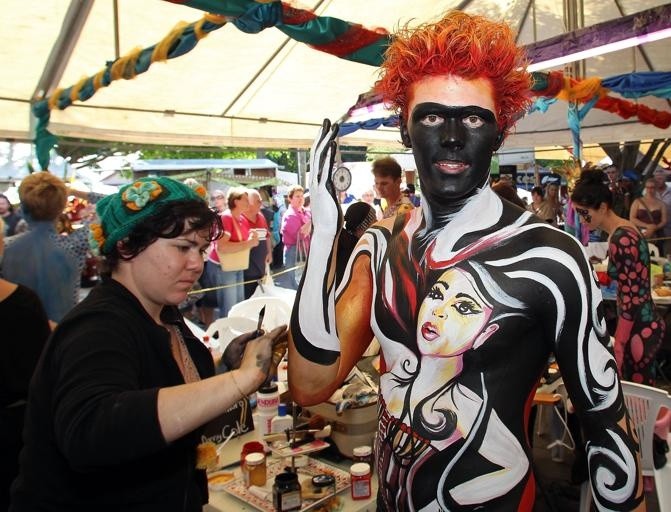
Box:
[533,362,562,436]
[202,426,377,512]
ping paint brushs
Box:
[291,455,296,472]
[214,430,237,454]
[256,305,265,335]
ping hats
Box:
[344,202,376,233]
[97,177,206,255]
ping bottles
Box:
[311,474,335,505]
[257,384,293,441]
[241,441,266,485]
[351,445,374,500]
[272,472,301,510]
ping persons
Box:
[0,172,102,328]
[571,160,671,387]
[185,178,315,328]
[0,276,51,512]
[494,157,671,258]
[337,156,420,285]
[375,263,530,512]
[43,177,288,512]
[288,12,648,512]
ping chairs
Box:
[557,381,671,512]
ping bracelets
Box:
[227,368,247,398]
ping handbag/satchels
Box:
[294,231,308,287]
[216,214,250,272]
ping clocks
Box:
[333,166,352,192]
